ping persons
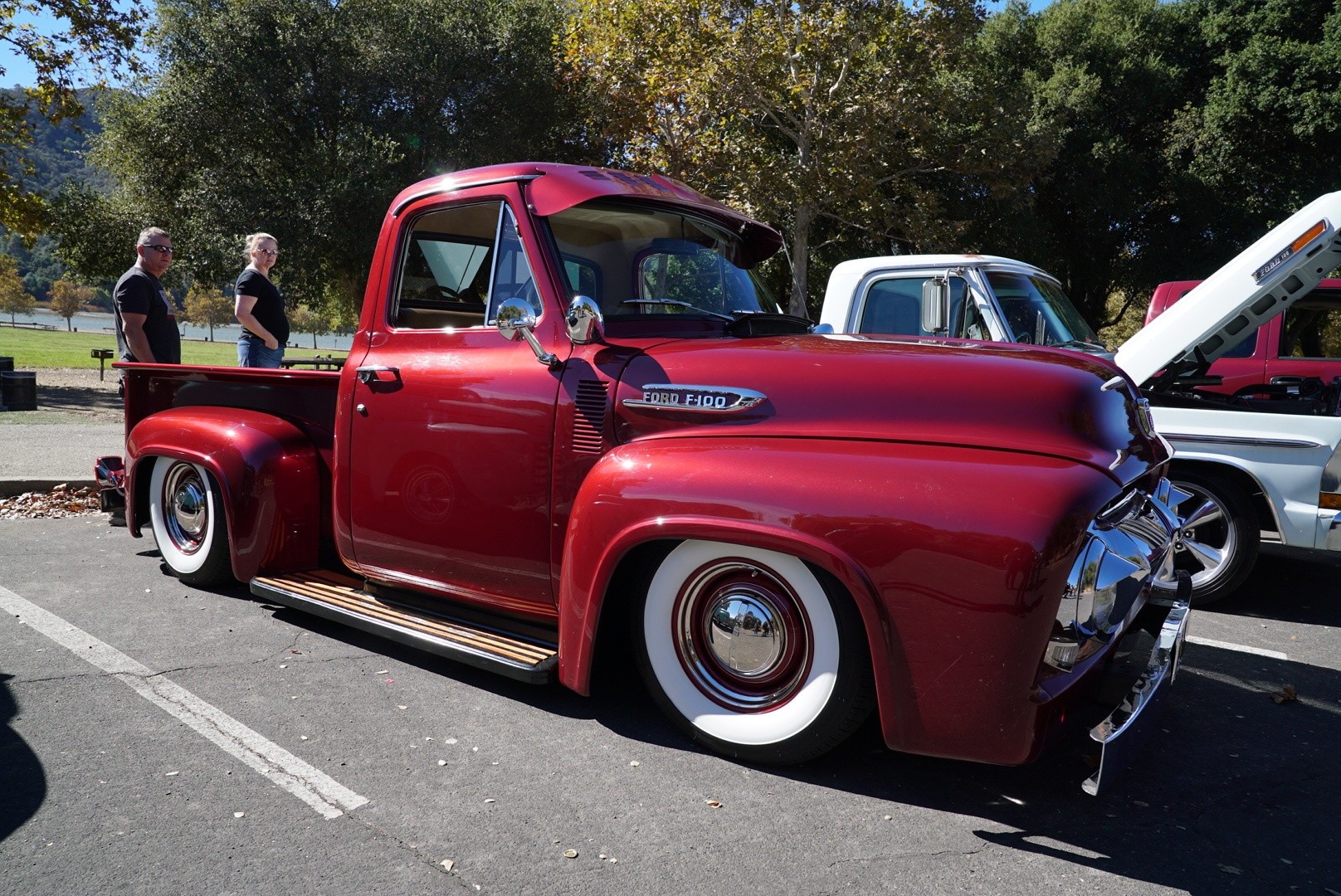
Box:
[109,226,183,529]
[234,232,291,369]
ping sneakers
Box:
[107,508,152,527]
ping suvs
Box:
[1133,267,1335,416]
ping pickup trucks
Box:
[95,156,1198,793]
[816,191,1335,596]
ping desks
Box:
[280,355,347,371]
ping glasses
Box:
[142,244,174,253]
[256,248,280,256]
